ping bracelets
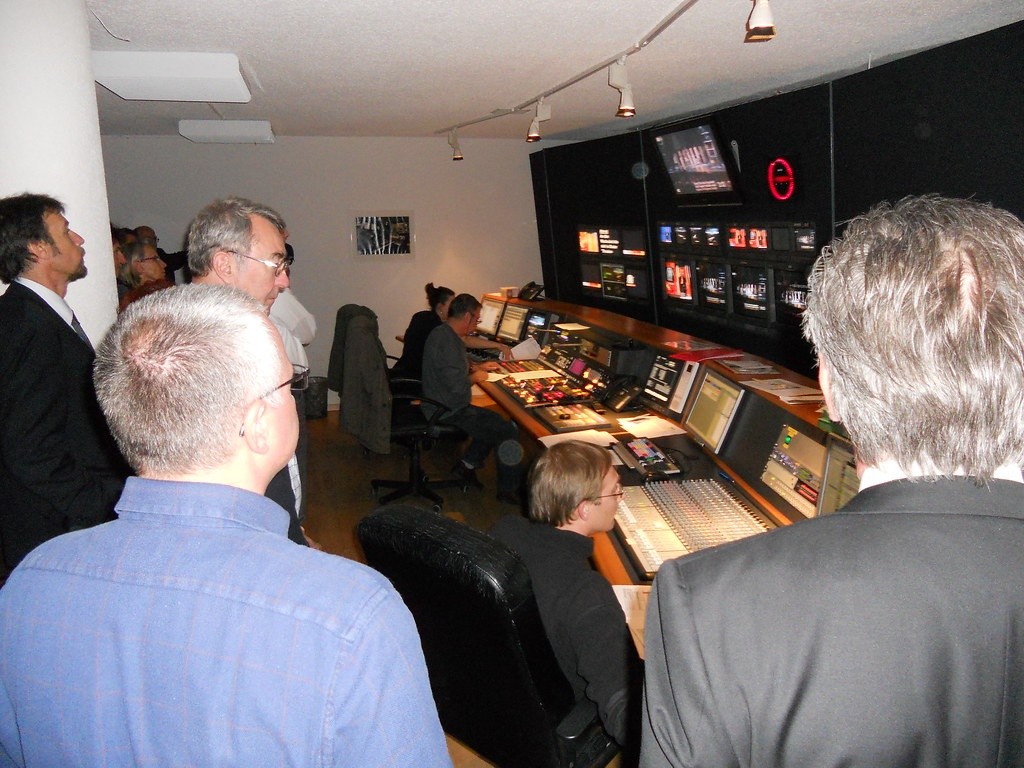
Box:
[469,363,475,372]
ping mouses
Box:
[639,471,670,483]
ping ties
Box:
[71,313,95,353]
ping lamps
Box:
[746,0,777,42]
[90,50,251,104]
[526,97,551,142]
[607,56,637,118]
[448,127,463,161]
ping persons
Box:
[109,220,186,312]
[638,192,1024,768]
[738,232,742,244]
[0,285,455,768]
[186,195,308,549]
[267,243,316,456]
[0,193,135,592]
[758,234,763,245]
[388,282,514,413]
[421,293,527,505]
[678,268,687,294]
[486,439,644,760]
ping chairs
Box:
[354,503,622,768]
[325,303,435,450]
[337,315,471,513]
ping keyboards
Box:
[615,436,680,476]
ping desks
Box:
[461,336,798,587]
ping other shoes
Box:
[454,461,485,490]
[496,491,525,505]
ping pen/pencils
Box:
[752,378,763,384]
[720,473,734,483]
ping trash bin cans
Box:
[304,376,328,419]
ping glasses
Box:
[472,314,482,325]
[239,364,310,436]
[227,250,290,276]
[140,256,160,263]
[144,238,159,244]
[578,483,624,504]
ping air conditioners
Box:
[179,118,276,145]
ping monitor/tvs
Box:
[654,220,825,333]
[579,225,648,302]
[652,117,744,207]
[815,432,861,516]
[476,297,552,346]
[683,366,746,455]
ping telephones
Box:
[601,376,644,413]
[518,281,544,301]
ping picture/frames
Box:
[350,209,416,261]
